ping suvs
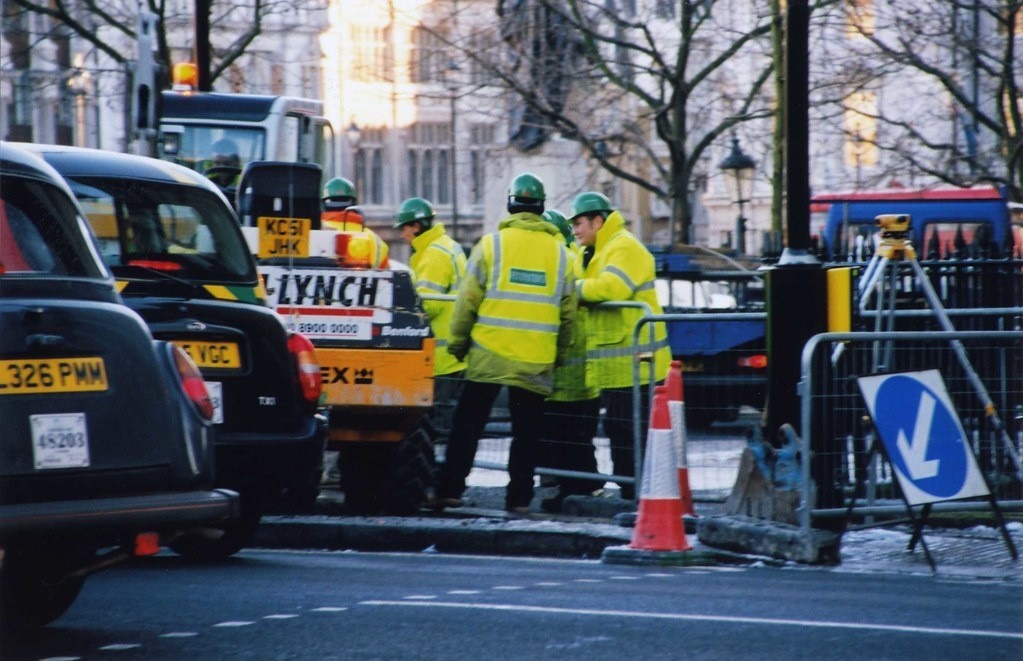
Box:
[0,142,328,628]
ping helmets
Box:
[320,177,356,200]
[568,192,615,224]
[507,173,546,201]
[540,209,574,245]
[393,198,436,228]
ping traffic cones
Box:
[602,386,719,566]
[613,358,706,534]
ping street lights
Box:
[717,132,755,256]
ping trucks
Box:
[66,63,436,509]
[640,185,1017,430]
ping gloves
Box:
[447,343,469,362]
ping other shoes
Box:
[317,464,342,489]
[504,501,528,513]
[419,489,465,508]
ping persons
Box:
[320,177,390,270]
[439,173,577,512]
[203,139,243,187]
[392,197,468,420]
[540,192,672,511]
